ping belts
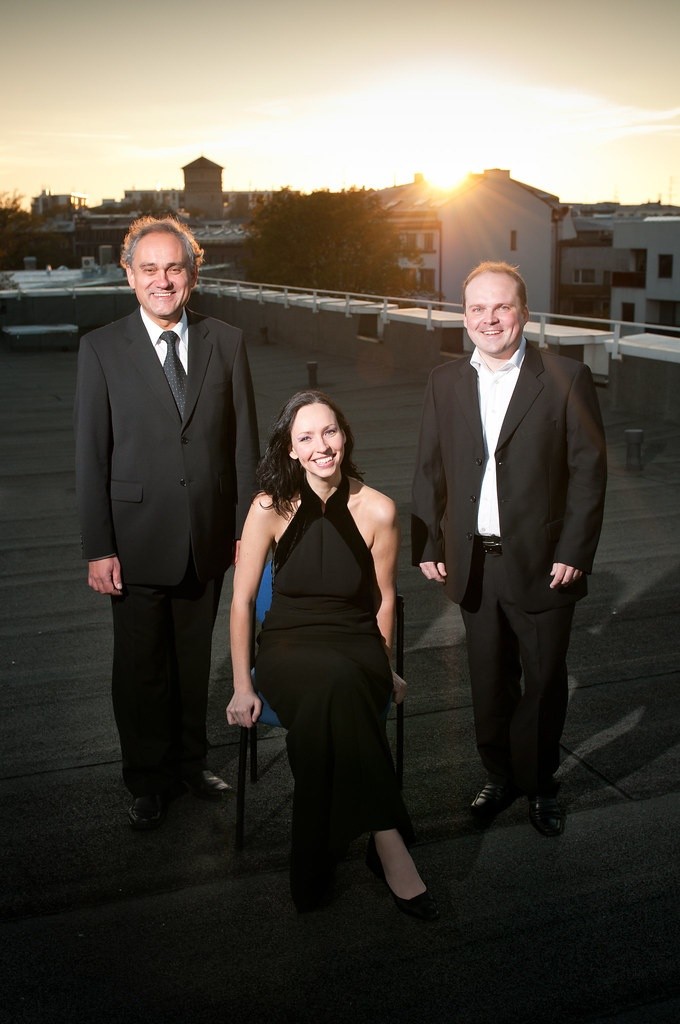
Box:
[474,541,502,555]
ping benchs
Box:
[2,323,79,349]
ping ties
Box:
[159,331,187,421]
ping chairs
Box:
[235,559,406,850]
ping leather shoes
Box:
[127,794,166,828]
[366,833,441,922]
[190,768,234,801]
[527,793,565,837]
[470,781,510,816]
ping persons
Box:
[73,215,262,832]
[411,260,608,839]
[227,391,436,921]
